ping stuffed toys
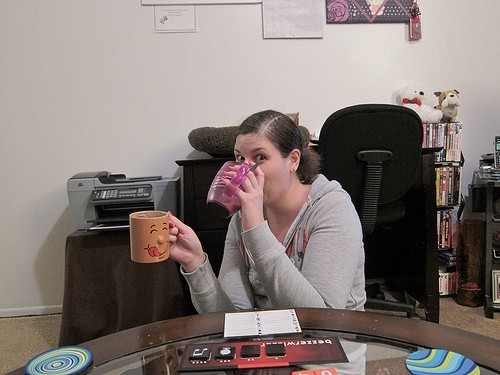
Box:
[434,89,459,122]
[401,85,443,123]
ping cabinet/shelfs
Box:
[423,124,500,319]
[175,158,235,316]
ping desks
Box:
[3,307,500,375]
[59,230,185,347]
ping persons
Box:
[166,110,367,375]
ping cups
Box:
[206,161,258,218]
[129,210,178,263]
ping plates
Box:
[406,349,481,375]
[24,346,93,375]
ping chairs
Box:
[317,104,423,319]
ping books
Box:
[224,309,302,339]
[422,122,462,295]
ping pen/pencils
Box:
[249,163,259,172]
